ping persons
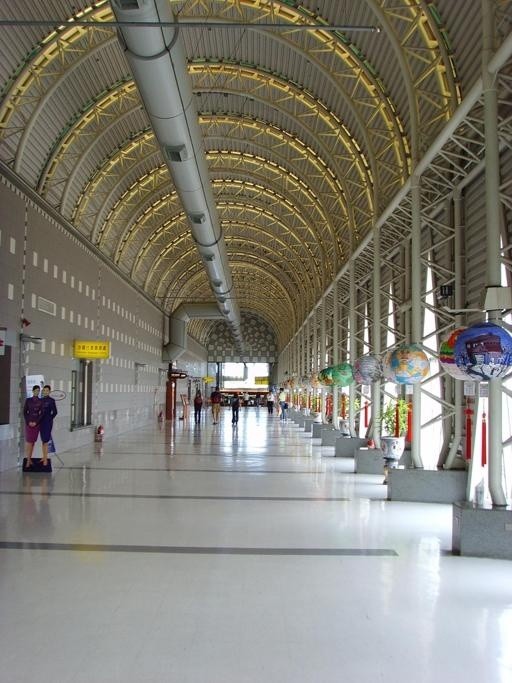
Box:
[194,389,204,424]
[24,384,45,468]
[255,392,260,408]
[244,391,249,406]
[211,424,219,445]
[210,386,223,424]
[231,425,239,462]
[266,390,274,413]
[40,384,57,466]
[229,392,242,426]
[239,392,243,408]
[277,387,285,419]
[193,424,202,448]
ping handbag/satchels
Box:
[196,397,202,404]
[233,400,240,410]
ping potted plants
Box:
[285,393,410,460]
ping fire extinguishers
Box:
[160,410,164,417]
[98,424,104,435]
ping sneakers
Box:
[232,421,238,425]
[195,420,201,424]
[213,421,218,424]
